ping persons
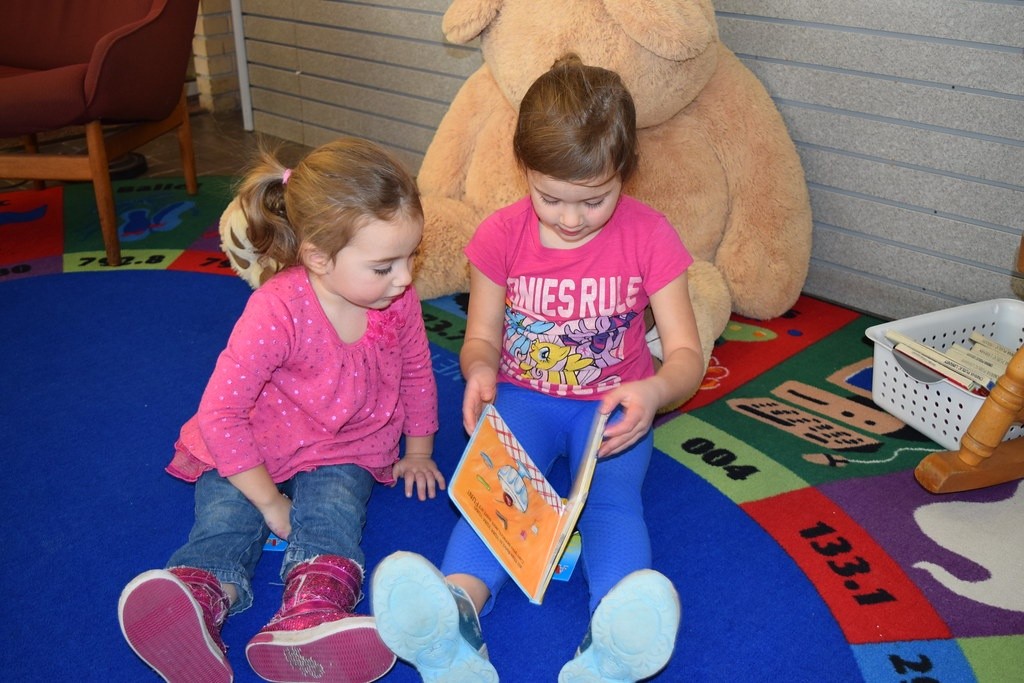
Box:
[368,59,702,683]
[118,139,444,683]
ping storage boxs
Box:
[862,297,1024,451]
[0,180,64,269]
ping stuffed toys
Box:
[217,0,814,377]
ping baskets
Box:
[864,299,1024,453]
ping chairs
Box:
[0,0,199,269]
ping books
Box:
[885,328,1018,395]
[448,403,609,604]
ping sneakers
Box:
[556,569,681,683]
[371,550,499,683]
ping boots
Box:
[118,567,234,683]
[243,554,395,683]
